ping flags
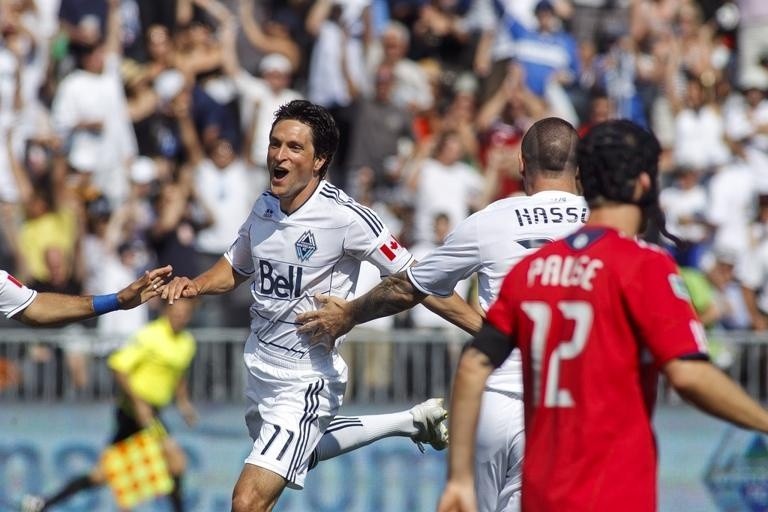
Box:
[98,426,177,512]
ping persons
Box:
[0,0,767,408]
[20,277,202,512]
[155,98,489,512]
[293,116,592,512]
[0,263,175,328]
[436,117,767,512]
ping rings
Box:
[152,284,158,289]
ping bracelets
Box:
[90,293,122,318]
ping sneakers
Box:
[411,396,450,454]
